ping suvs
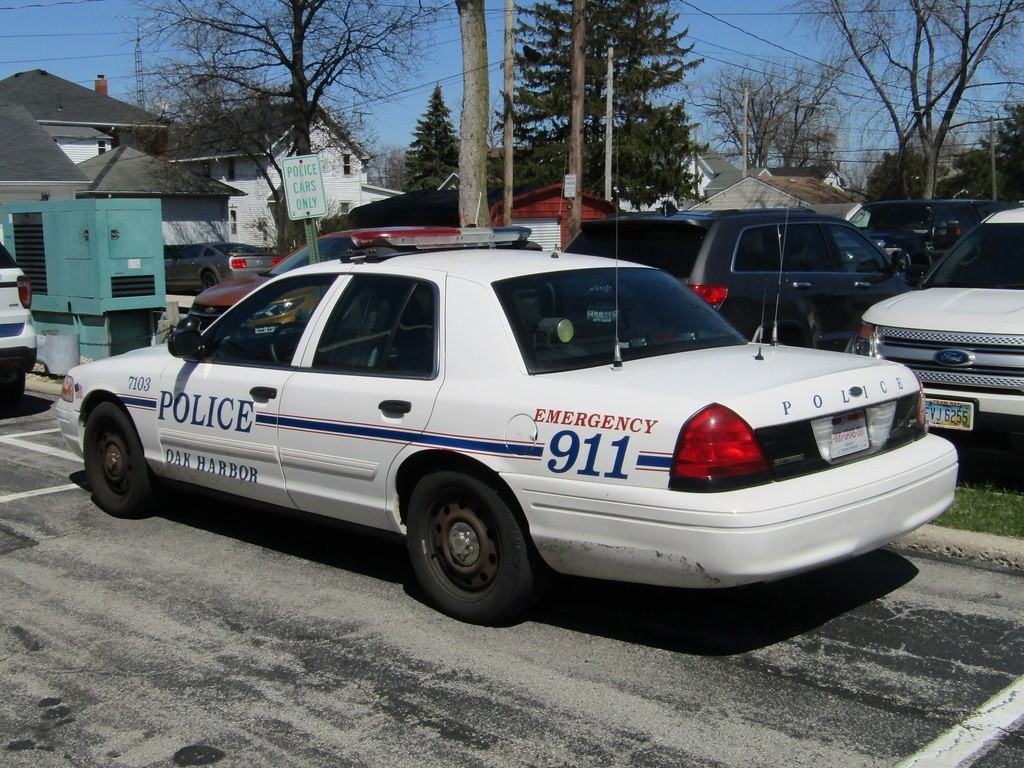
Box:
[842,205,1024,438]
[830,199,1024,288]
[541,207,918,351]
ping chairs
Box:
[332,292,389,370]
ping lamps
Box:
[41,191,52,202]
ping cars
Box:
[0,242,39,408]
[188,228,359,345]
[56,221,960,627]
